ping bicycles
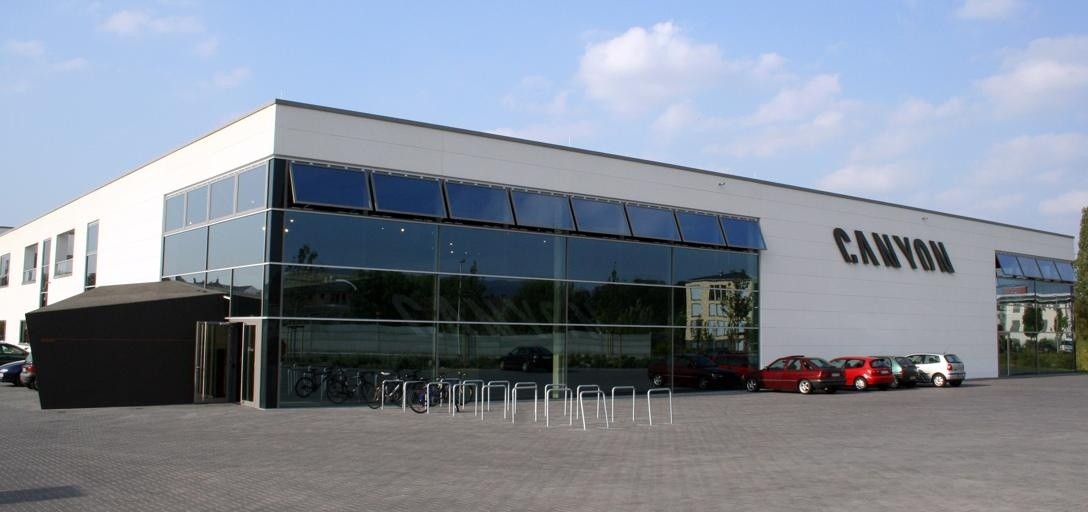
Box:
[294,366,376,405]
[366,370,473,414]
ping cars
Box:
[497,345,553,371]
[0,343,38,391]
[648,353,757,390]
[745,353,966,394]
